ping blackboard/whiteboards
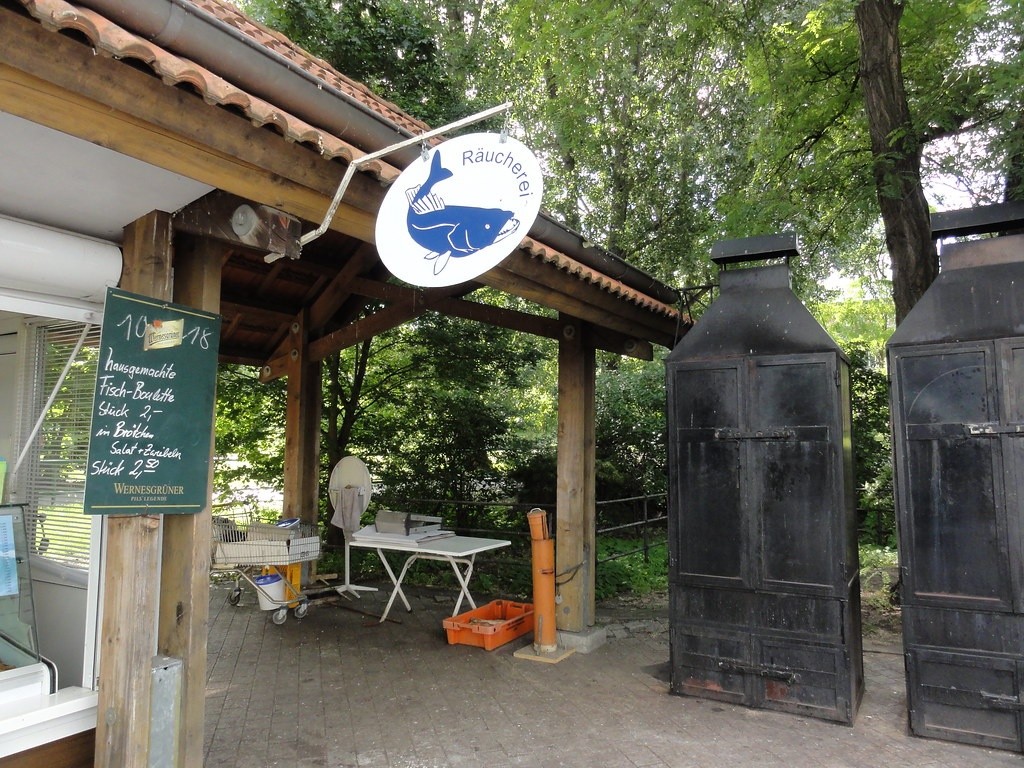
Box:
[82,286,223,515]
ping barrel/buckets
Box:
[274,518,301,539]
[254,574,285,611]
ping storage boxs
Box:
[262,562,302,608]
[443,600,534,651]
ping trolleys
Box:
[204,503,326,625]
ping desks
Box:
[348,537,511,624]
